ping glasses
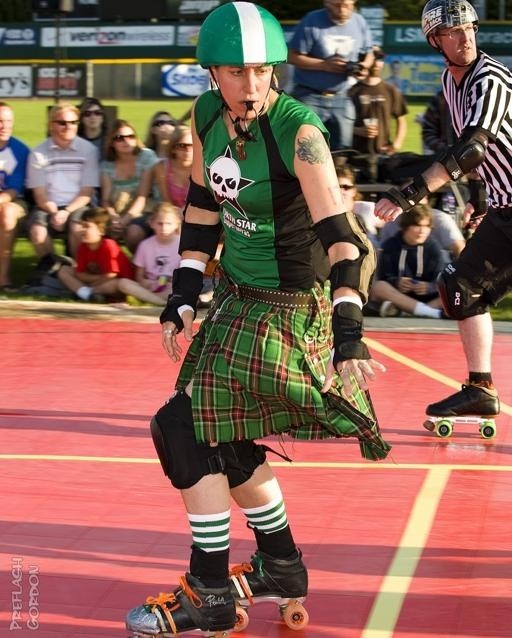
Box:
[48,119,81,126]
[155,119,177,126]
[174,142,193,153]
[82,109,104,117]
[112,134,136,142]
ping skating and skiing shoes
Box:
[125,571,250,638]
[228,548,309,631]
[423,381,501,440]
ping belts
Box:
[217,260,328,309]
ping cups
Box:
[364,117,379,138]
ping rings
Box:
[338,369,348,375]
[164,330,173,334]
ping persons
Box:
[124,1,390,633]
[1,1,482,320]
[373,0,511,418]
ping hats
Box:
[359,43,386,60]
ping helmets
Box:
[420,0,480,38]
[194,1,289,67]
